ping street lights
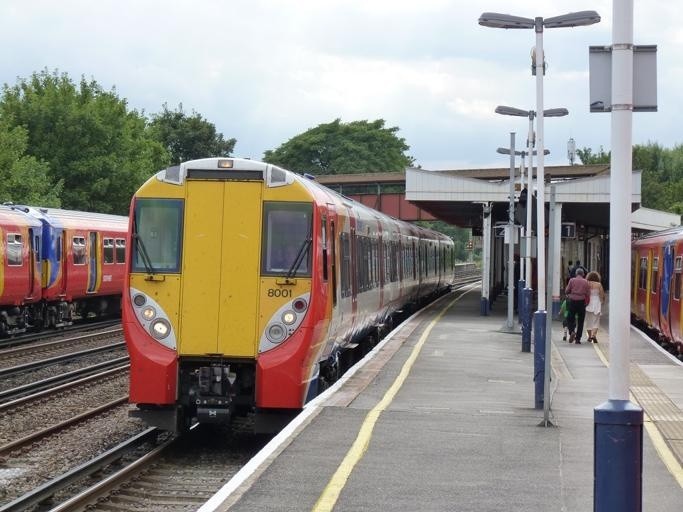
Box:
[495,105,568,352]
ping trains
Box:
[0,156,453,434]
[602,226,683,362]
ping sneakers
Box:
[562,331,598,344]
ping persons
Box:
[564,267,591,345]
[566,260,587,280]
[565,260,573,275]
[585,270,605,343]
[558,298,577,341]
